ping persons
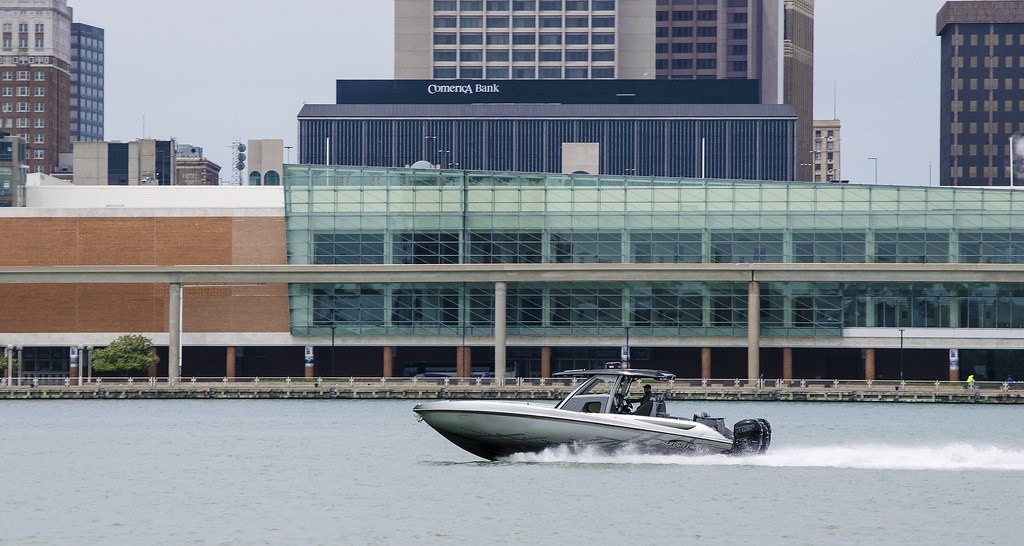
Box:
[628,384,652,414]
[965,374,976,388]
[1006,374,1017,389]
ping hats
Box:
[643,384,651,389]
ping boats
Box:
[413,368,772,462]
